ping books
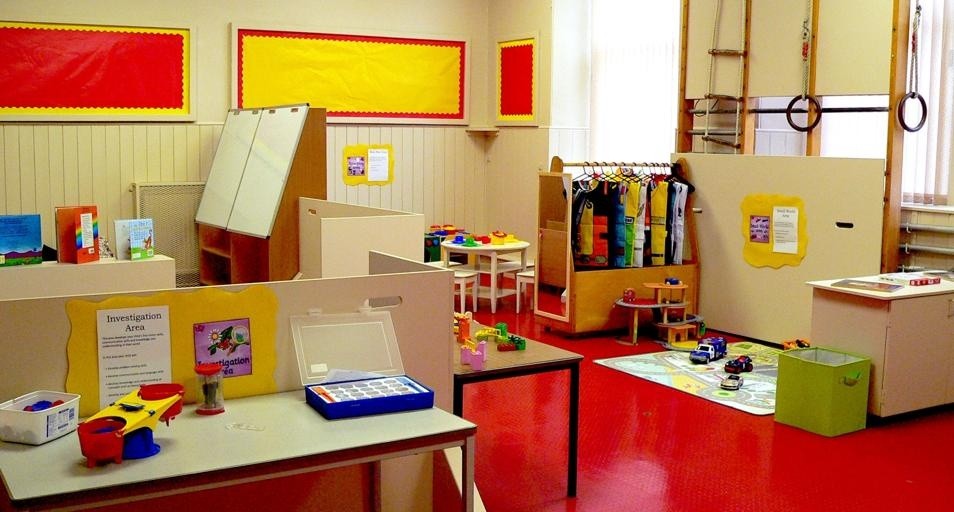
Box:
[55,204,100,265]
[880,275,941,286]
[0,213,42,267]
[112,218,155,261]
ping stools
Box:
[426,260,461,268]
[503,260,533,279]
[452,271,478,314]
[516,271,534,310]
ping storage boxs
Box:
[774,347,871,438]
[0,390,81,446]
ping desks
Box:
[0,384,477,511]
[440,236,532,312]
[452,315,585,498]
[808,270,953,419]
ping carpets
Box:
[592,340,784,416]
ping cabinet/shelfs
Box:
[196,223,268,288]
[616,281,694,345]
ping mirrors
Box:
[533,171,572,321]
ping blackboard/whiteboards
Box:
[191,103,310,238]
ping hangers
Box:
[573,160,696,198]
